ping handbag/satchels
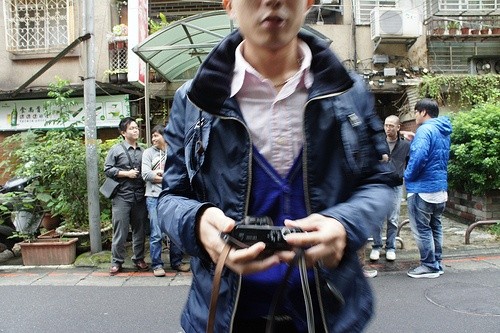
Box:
[99,177,120,199]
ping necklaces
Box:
[274,54,301,87]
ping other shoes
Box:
[110,265,122,276]
[172,264,190,271]
[386,250,396,260]
[362,268,377,277]
[153,267,165,277]
[133,261,148,272]
[370,250,380,260]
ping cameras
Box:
[224,225,303,257]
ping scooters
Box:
[0,173,42,258]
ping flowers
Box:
[105,23,128,44]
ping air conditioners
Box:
[370,7,422,40]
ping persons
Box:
[358,115,415,278]
[142,125,191,277]
[403,99,452,278]
[157,0,395,333]
[104,117,149,276]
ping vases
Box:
[109,43,114,50]
[116,42,124,49]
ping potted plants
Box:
[104,68,128,83]
[148,14,192,84]
[0,130,147,265]
[433,16,500,34]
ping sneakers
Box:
[407,265,444,278]
